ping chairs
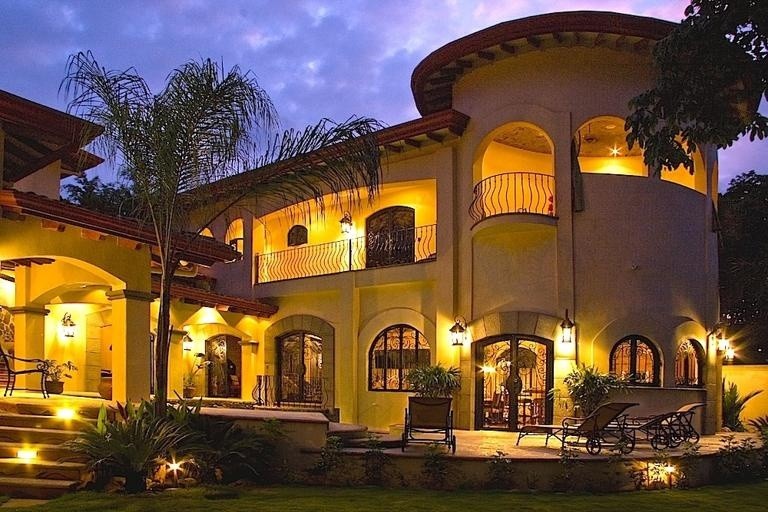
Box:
[398,395,457,457]
[541,398,706,450]
[515,400,640,455]
[0,343,51,399]
[484,389,502,424]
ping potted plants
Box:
[182,373,197,398]
[40,359,76,393]
[57,395,198,493]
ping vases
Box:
[98,376,112,401]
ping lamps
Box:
[450,312,468,348]
[339,209,354,235]
[60,311,77,337]
[560,308,575,343]
